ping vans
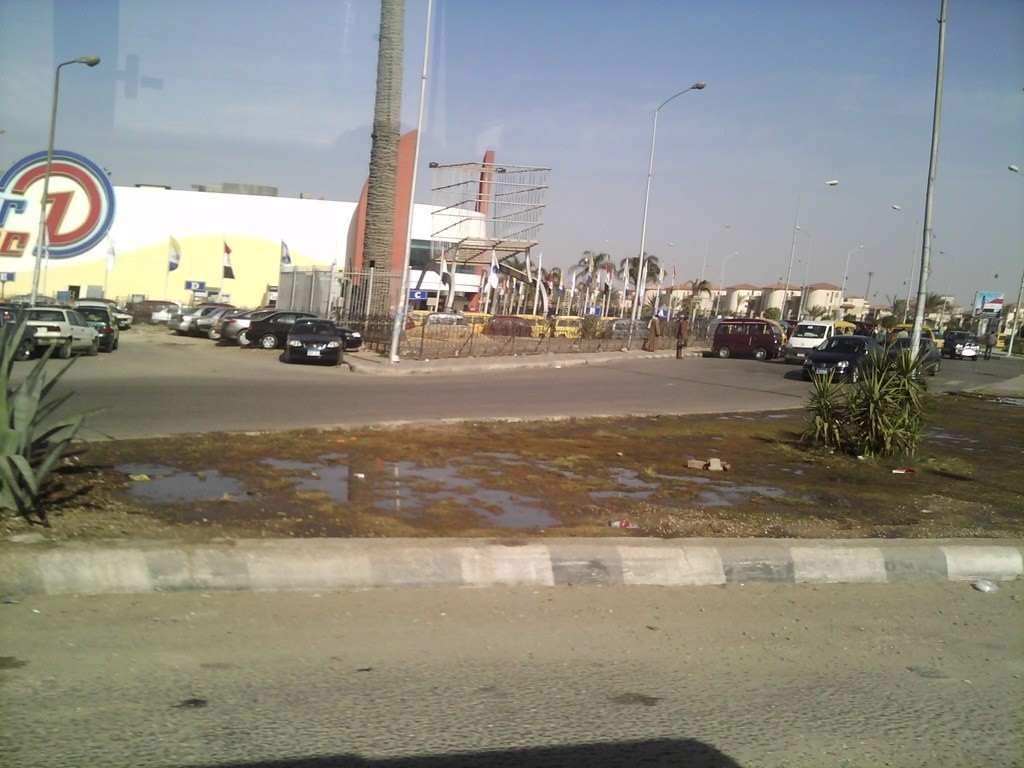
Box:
[789,317,1006,362]
[128,300,178,326]
[71,301,120,352]
[406,308,651,340]
[706,316,797,360]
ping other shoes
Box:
[649,350,654,352]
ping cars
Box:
[245,311,362,351]
[189,307,244,339]
[109,306,132,329]
[221,308,289,347]
[285,317,343,366]
[150,306,181,325]
[22,308,100,358]
[802,335,941,382]
[0,307,39,361]
[169,306,224,335]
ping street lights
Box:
[29,56,101,311]
[893,204,918,323]
[1006,164,1024,358]
[627,80,707,350]
[779,180,839,322]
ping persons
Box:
[982,296,986,310]
[836,325,908,346]
[676,317,689,359]
[646,314,660,351]
[985,331,996,353]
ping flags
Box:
[499,255,677,303]
[169,236,181,271]
[490,252,500,289]
[223,243,235,279]
[441,252,448,285]
[281,242,291,271]
[107,239,115,269]
